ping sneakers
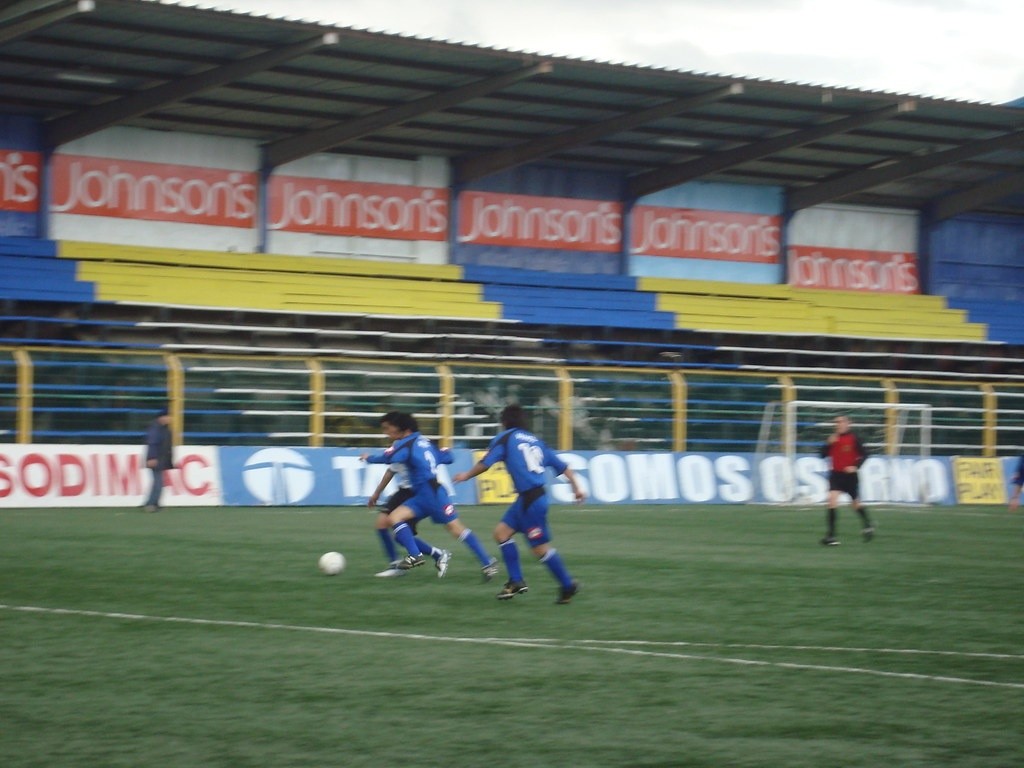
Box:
[396,552,427,570]
[554,582,579,604]
[374,559,408,576]
[435,549,452,578]
[480,556,499,581]
[496,577,529,601]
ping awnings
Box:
[0,0,1024,220]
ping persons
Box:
[327,410,500,583]
[449,404,590,604]
[145,411,175,512]
[1008,453,1024,512]
[818,415,879,546]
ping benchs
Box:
[0,239,1024,455]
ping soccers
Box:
[319,550,346,576]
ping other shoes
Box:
[863,525,874,542]
[143,505,158,512]
[820,536,841,546]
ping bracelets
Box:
[1011,495,1019,499]
